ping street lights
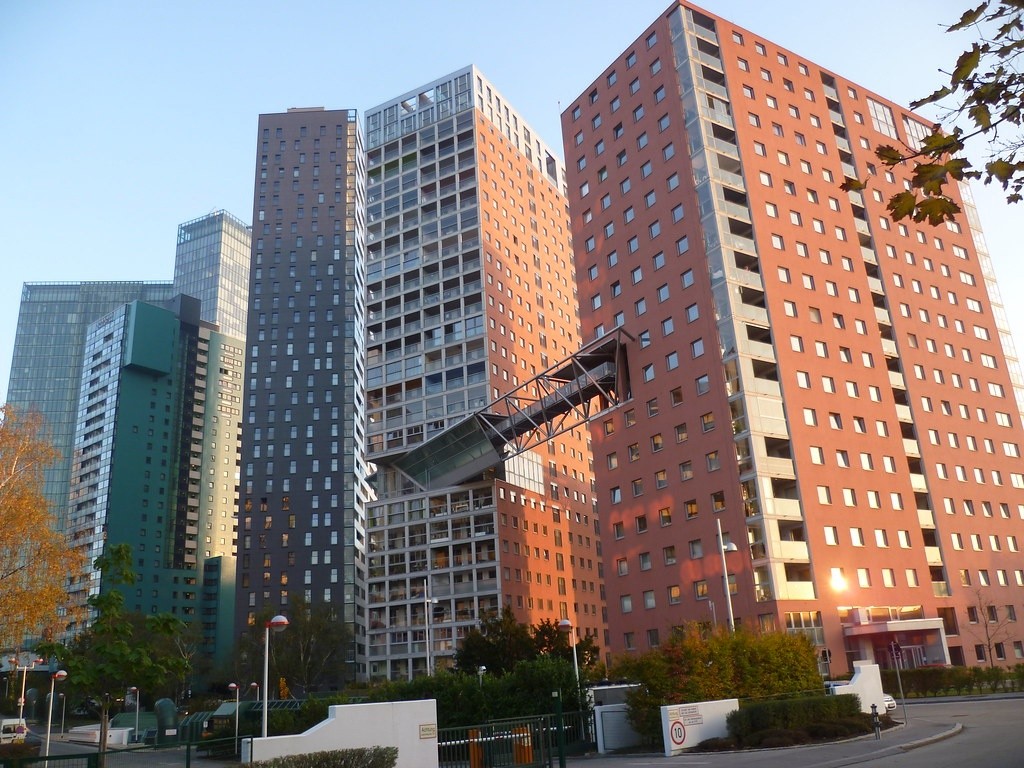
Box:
[558,620,586,741]
[59,693,66,739]
[228,683,240,754]
[44,670,68,768]
[424,578,439,677]
[250,682,260,701]
[8,658,44,727]
[261,615,291,737]
[717,518,739,632]
[131,687,139,740]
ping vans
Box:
[0,718,27,745]
